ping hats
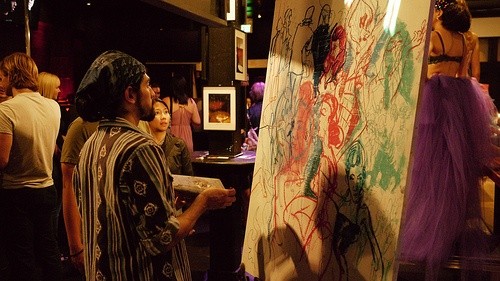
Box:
[77,49,146,123]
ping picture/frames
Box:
[233,28,248,82]
[201,84,238,133]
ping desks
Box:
[190,148,256,166]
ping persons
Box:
[0,51,268,281]
[400,0,500,281]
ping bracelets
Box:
[70,249,84,257]
